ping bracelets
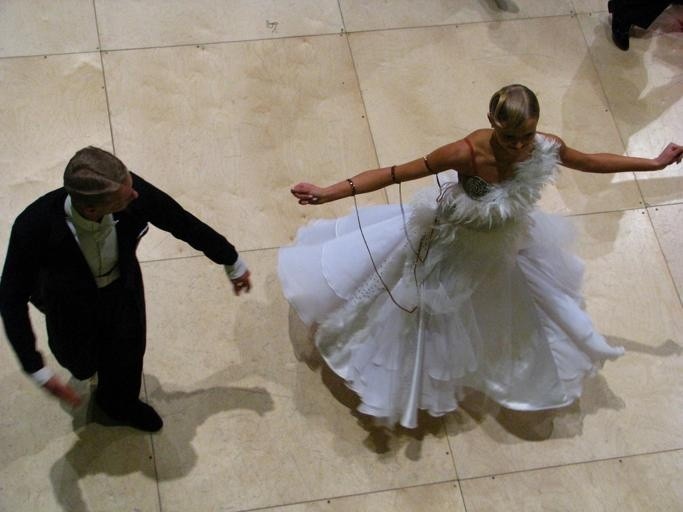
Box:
[345,178,356,197]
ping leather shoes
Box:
[92,389,163,432]
[612,12,631,51]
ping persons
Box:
[0,146,254,434]
[273,80,682,435]
[606,0,672,50]
